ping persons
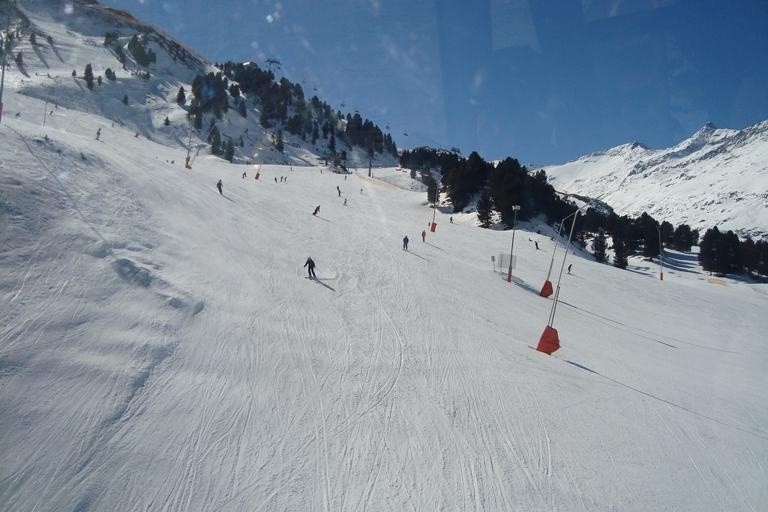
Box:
[535,241,539,249]
[304,256,316,277]
[450,217,453,223]
[313,205,320,216]
[422,230,426,242]
[403,236,409,251]
[568,264,572,274]
[217,180,223,195]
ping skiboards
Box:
[304,276,317,280]
